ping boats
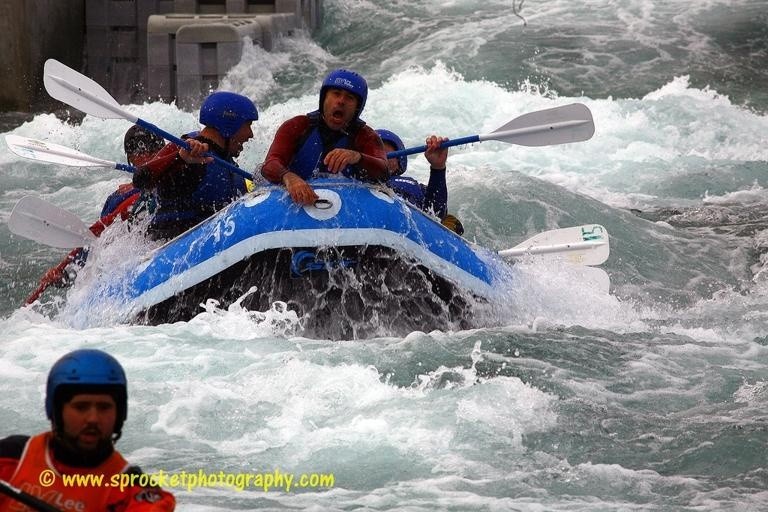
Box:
[73,178,517,333]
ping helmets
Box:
[124,125,166,154]
[319,70,368,124]
[200,92,259,140]
[44,348,127,421]
[374,129,407,177]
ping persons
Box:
[260,69,395,205]
[1,348,176,512]
[131,91,259,249]
[43,125,164,291]
[375,128,466,237]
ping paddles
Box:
[5,133,137,173]
[7,195,98,251]
[496,224,610,266]
[386,104,595,160]
[43,60,256,179]
